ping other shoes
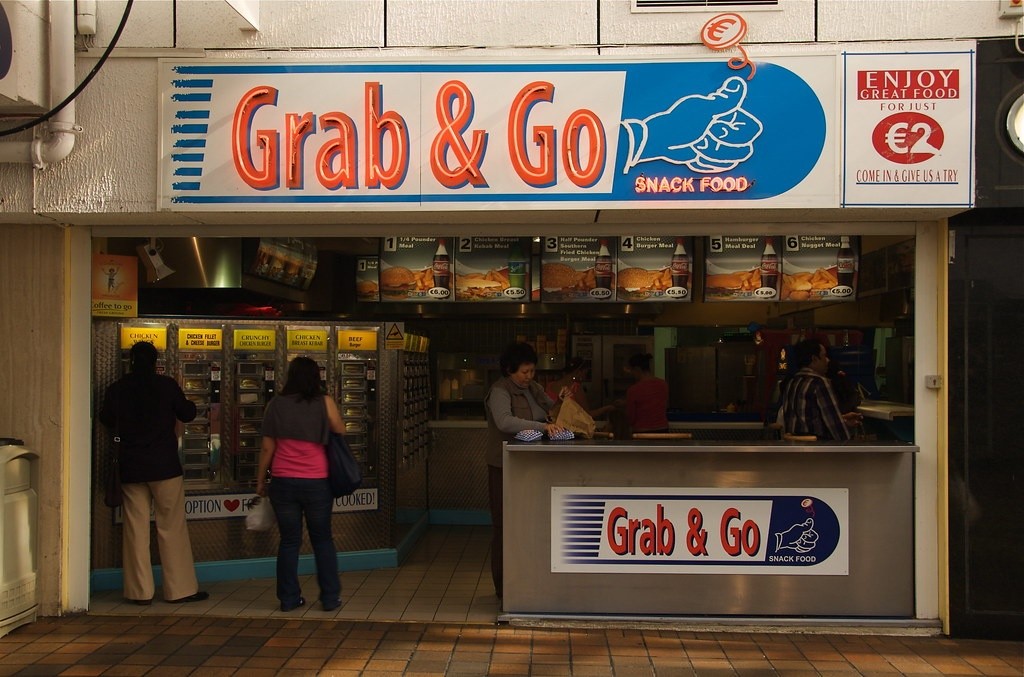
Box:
[294,597,305,607]
[132,599,152,605]
[335,598,342,606]
[167,592,209,603]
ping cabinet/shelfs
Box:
[435,353,567,420]
[118,317,429,494]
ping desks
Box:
[503,438,920,614]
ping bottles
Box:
[670,238,690,299]
[507,240,527,300]
[432,239,451,299]
[760,238,779,299]
[595,238,612,300]
[836,236,855,297]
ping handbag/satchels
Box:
[104,435,122,507]
[327,431,362,497]
[546,388,596,439]
[244,492,277,532]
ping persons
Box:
[546,358,620,418]
[483,344,574,599]
[257,357,348,612]
[99,343,209,606]
[783,340,862,439]
[613,353,671,432]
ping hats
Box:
[565,357,586,376]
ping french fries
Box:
[735,270,761,290]
[783,269,838,290]
[648,269,672,291]
[412,269,434,290]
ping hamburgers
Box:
[381,266,417,299]
[542,263,579,301]
[707,275,742,299]
[618,268,653,300]
[358,280,377,298]
